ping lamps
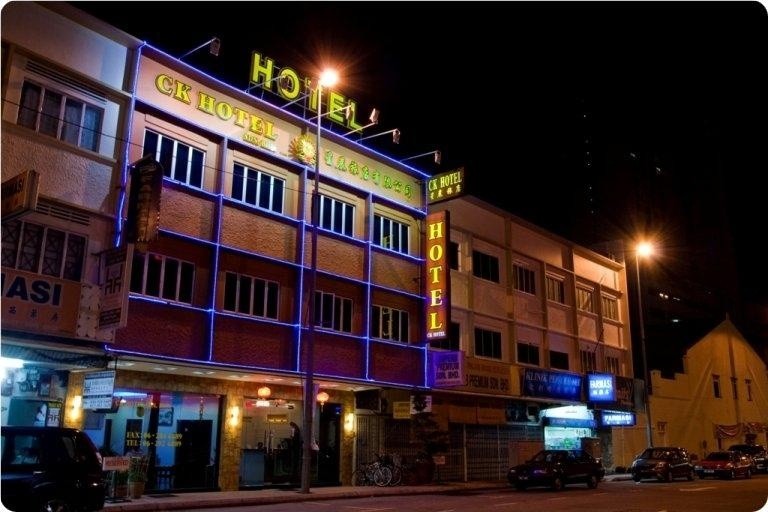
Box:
[309,74,448,166]
[180,37,220,62]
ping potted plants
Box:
[126,472,149,499]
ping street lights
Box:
[298,65,341,488]
[634,243,654,450]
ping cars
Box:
[630,440,768,482]
[508,450,607,490]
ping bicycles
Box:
[350,453,404,488]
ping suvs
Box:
[1,425,107,511]
